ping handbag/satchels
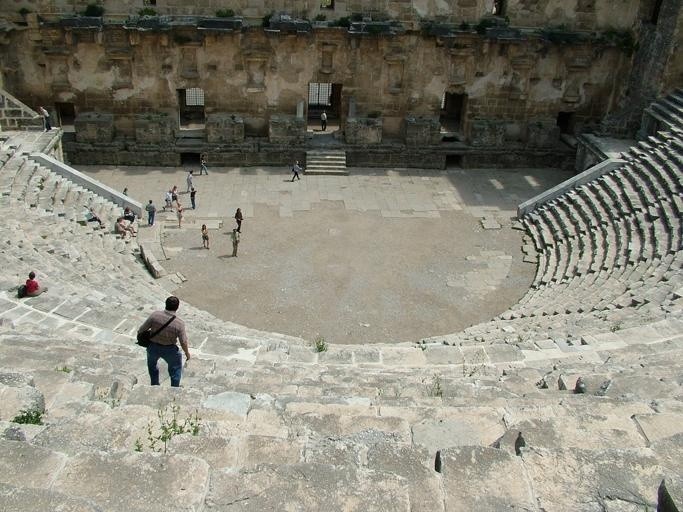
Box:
[136,330,150,348]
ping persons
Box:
[25,271,49,297]
[199,224,211,250]
[199,153,210,175]
[233,207,245,233]
[228,228,242,258]
[38,106,53,130]
[114,169,198,240]
[86,207,106,228]
[137,296,191,388]
[290,160,303,182]
[319,109,329,131]
